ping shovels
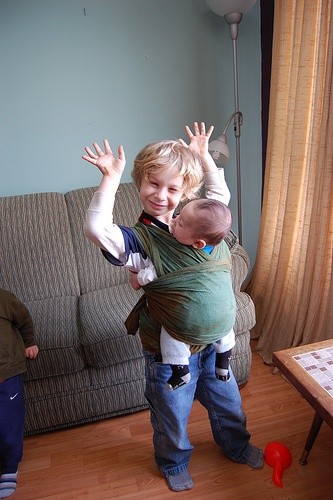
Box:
[264,441,293,488]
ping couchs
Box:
[0,182,257,438]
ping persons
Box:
[80,121,265,493]
[0,288,39,498]
[129,198,237,391]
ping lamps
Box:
[204,0,256,247]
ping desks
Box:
[271,338,333,466]
